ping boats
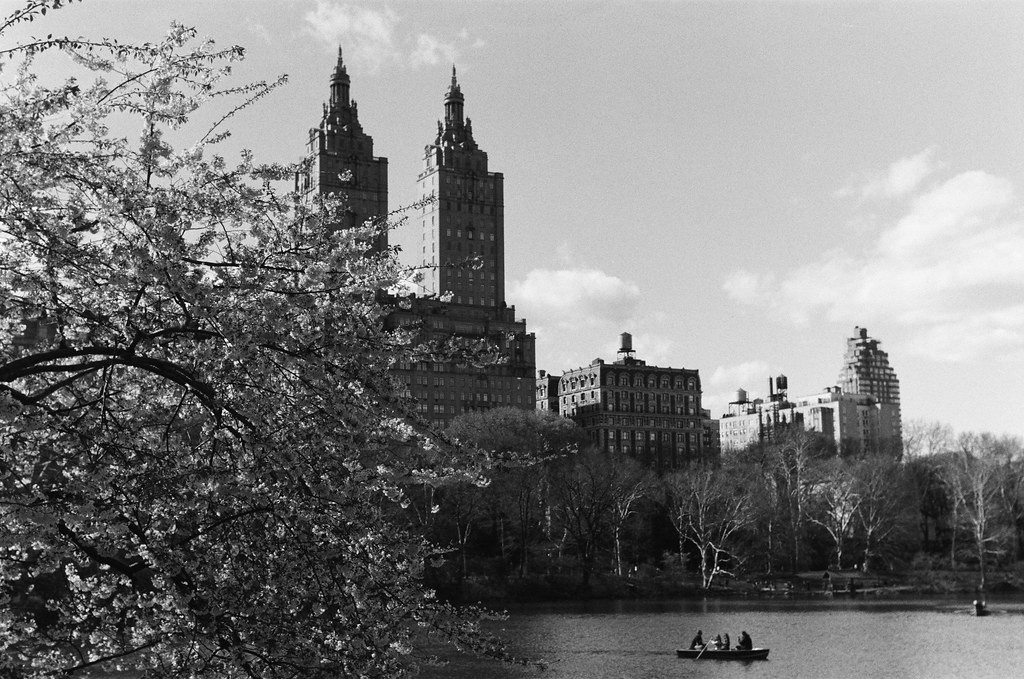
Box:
[676,648,770,660]
[967,609,991,617]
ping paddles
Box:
[954,608,975,614]
[984,608,1007,613]
[696,637,712,659]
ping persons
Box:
[689,630,707,649]
[715,633,730,650]
[736,631,752,650]
